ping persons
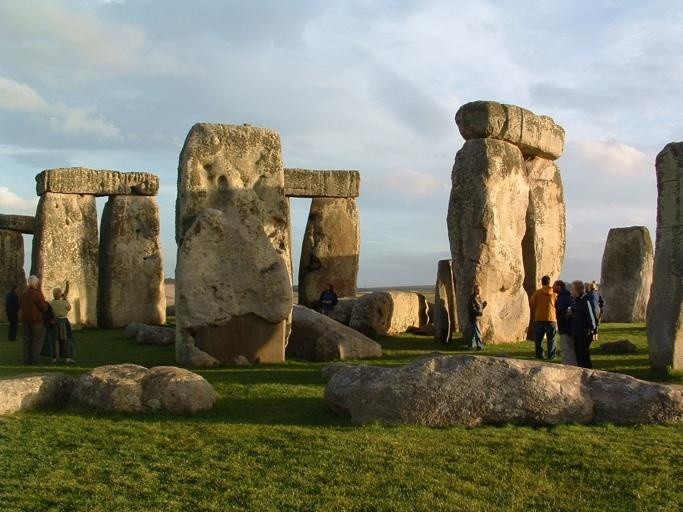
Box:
[468,284,488,350]
[47,280,75,363]
[530,276,606,367]
[5,286,21,343]
[21,276,50,365]
[318,282,337,313]
